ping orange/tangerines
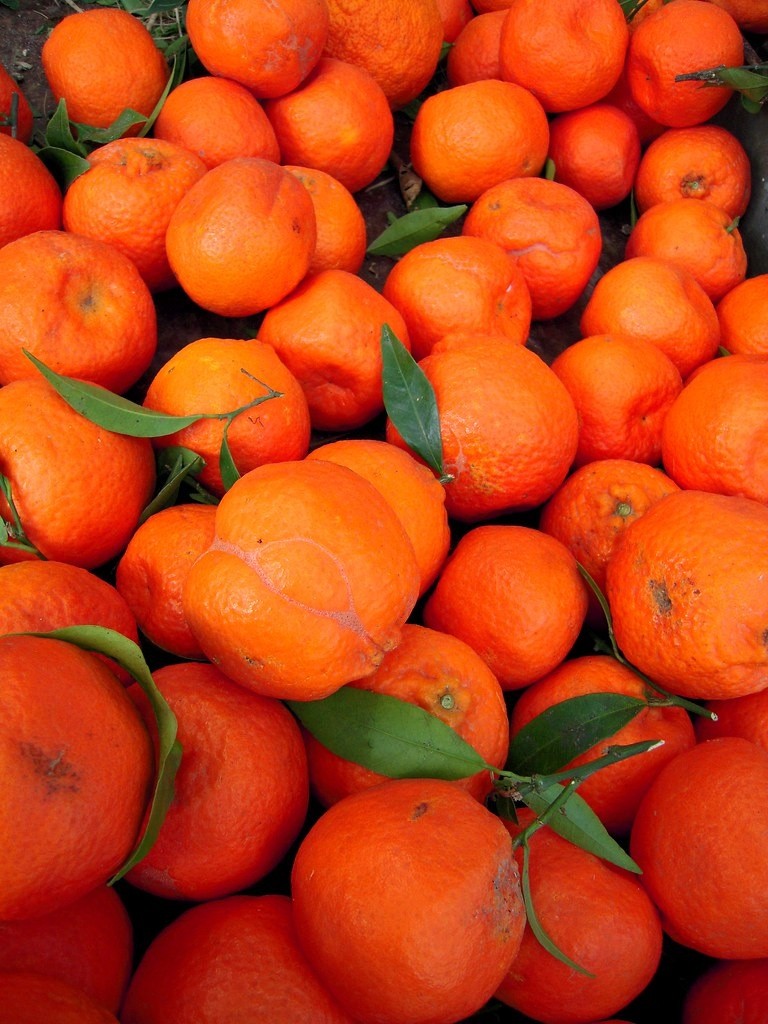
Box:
[0,0,768,1024]
